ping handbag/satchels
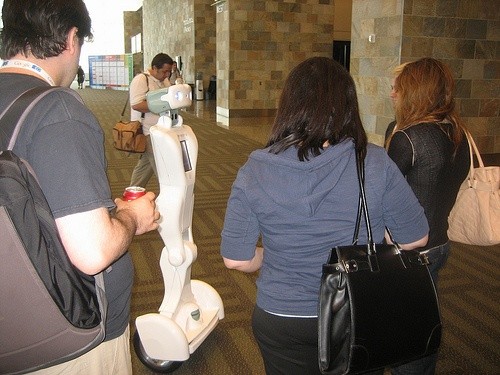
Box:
[112,121,148,154]
[317,144,444,375]
[447,127,500,247]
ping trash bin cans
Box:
[210,75,216,100]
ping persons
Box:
[382,57,471,375]
[220,57,430,375]
[130,52,182,189]
[78,66,84,89]
[0,0,161,375]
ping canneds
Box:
[122,186,146,201]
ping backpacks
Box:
[0,85,107,375]
